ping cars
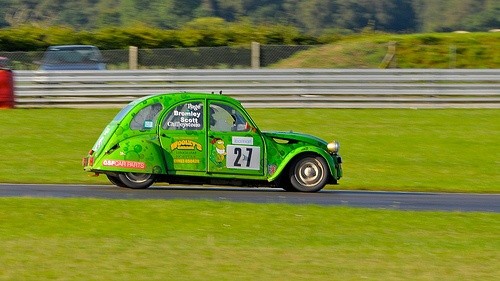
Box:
[40,46,108,73]
[84,90,345,192]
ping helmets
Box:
[199,106,217,126]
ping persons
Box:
[209,107,217,130]
